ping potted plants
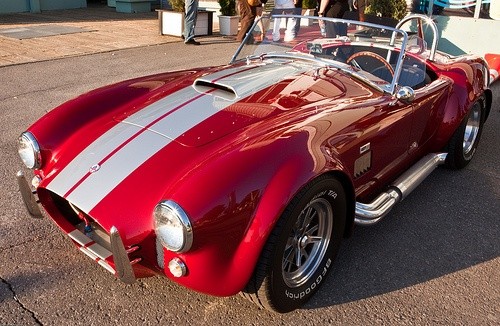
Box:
[299,0,317,26]
[153,0,216,39]
[353,0,419,48]
[217,0,240,36]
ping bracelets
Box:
[318,12,323,16]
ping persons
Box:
[181,0,200,45]
[352,0,365,31]
[272,0,297,42]
[318,0,350,37]
[236,0,267,45]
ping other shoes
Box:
[178,26,298,46]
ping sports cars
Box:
[15,13,491,311]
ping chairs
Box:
[372,63,431,89]
